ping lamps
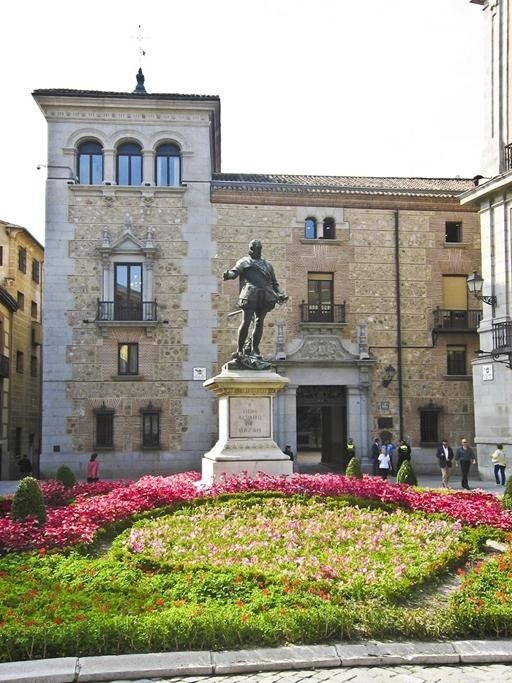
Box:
[466,270,497,308]
[384,365,396,385]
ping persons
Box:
[222,239,285,361]
[283,445,293,461]
[436,440,454,488]
[86,451,101,481]
[491,442,506,487]
[345,437,355,468]
[18,453,33,479]
[370,437,412,482]
[454,438,477,489]
